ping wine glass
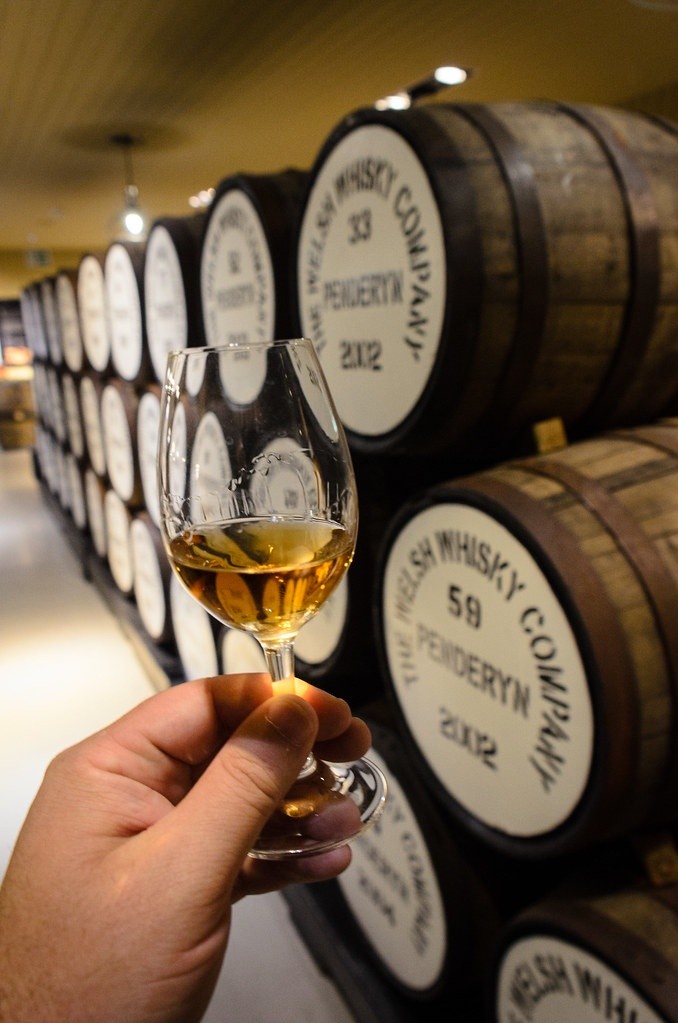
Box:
[155,338,387,861]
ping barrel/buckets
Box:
[20,96,678,1022]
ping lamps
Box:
[116,140,143,233]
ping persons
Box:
[0,673,372,1023]
